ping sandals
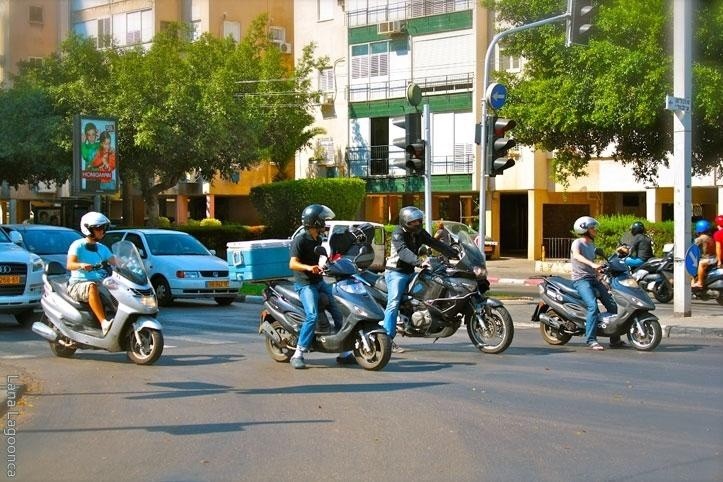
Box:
[336,353,359,365]
[290,356,306,369]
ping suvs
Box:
[0,227,47,327]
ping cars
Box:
[0,223,110,284]
[100,229,244,307]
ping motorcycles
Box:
[596,240,673,306]
[530,246,663,352]
[327,219,514,354]
[30,239,164,365]
[249,244,393,371]
[653,242,723,310]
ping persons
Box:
[289,203,358,368]
[432,221,451,247]
[39,209,59,227]
[383,206,462,353]
[66,212,123,337]
[569,215,627,350]
[691,215,723,289]
[616,221,651,269]
[83,122,115,189]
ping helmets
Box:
[695,220,710,236]
[573,216,599,237]
[715,215,723,227]
[629,222,643,236]
[80,211,112,237]
[398,206,423,235]
[302,204,335,231]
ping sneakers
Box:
[102,318,114,338]
[391,341,404,353]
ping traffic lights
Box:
[485,115,517,178]
[404,138,425,177]
[566,0,598,46]
[391,111,420,179]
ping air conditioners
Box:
[375,20,400,36]
[276,41,291,55]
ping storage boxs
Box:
[224,238,295,282]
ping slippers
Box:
[587,343,604,351]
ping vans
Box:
[286,220,385,273]
